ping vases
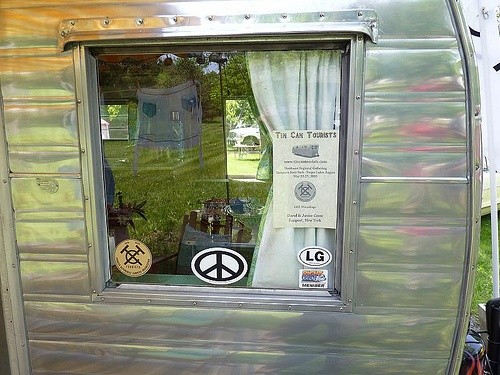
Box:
[107,218,126,227]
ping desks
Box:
[182,211,259,258]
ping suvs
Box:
[225,104,331,148]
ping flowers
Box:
[107,194,150,229]
